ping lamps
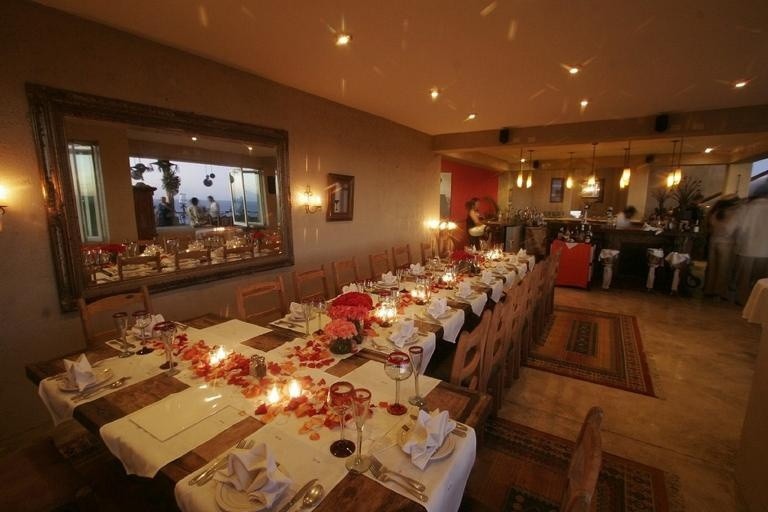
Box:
[666,138,683,186]
[618,147,631,189]
[587,143,599,187]
[296,184,322,215]
[565,152,574,189]
[516,147,535,189]
[204,164,215,187]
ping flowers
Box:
[669,175,705,210]
[649,187,672,203]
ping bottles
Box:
[249,355,266,376]
[558,224,592,244]
[471,255,481,275]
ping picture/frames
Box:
[578,178,603,203]
[549,177,564,202]
[325,172,355,221]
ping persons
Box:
[685,195,705,227]
[614,206,636,229]
[153,195,219,227]
[465,198,486,252]
[703,191,768,307]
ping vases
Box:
[680,206,692,220]
[659,201,664,209]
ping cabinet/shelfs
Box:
[133,183,158,239]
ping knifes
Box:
[277,475,318,511]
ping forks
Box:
[368,453,429,503]
[188,438,255,487]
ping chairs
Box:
[0,239,604,512]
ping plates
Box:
[57,372,112,391]
[285,311,310,321]
[216,479,265,511]
[399,421,454,459]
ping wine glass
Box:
[354,258,456,329]
[301,296,326,338]
[330,346,423,474]
[112,311,182,377]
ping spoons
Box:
[300,485,322,510]
[75,380,123,403]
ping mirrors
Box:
[26,80,295,314]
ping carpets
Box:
[521,305,665,400]
[458,416,685,512]
